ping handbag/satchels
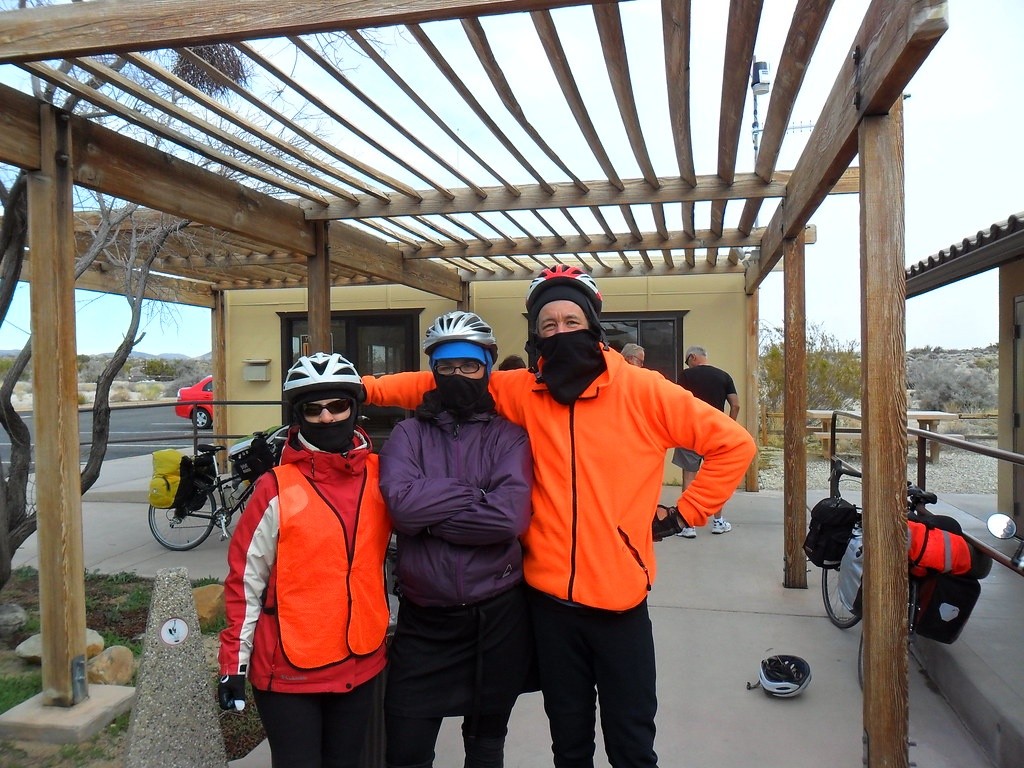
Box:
[905,512,994,643]
[184,453,215,509]
[149,449,194,509]
[838,520,867,619]
[802,497,857,569]
[672,447,702,472]
[233,438,273,481]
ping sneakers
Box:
[712,520,731,534]
[678,526,698,538]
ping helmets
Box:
[284,352,365,403]
[759,654,812,699]
[423,311,498,378]
[525,264,610,351]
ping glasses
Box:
[434,360,481,375]
[300,397,352,417]
[685,354,692,366]
[633,355,644,366]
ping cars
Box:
[175,375,213,429]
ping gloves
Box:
[651,505,687,542]
[217,675,246,712]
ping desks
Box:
[806,409,960,464]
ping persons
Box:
[377,311,533,768]
[621,343,644,368]
[671,346,740,537]
[218,352,393,768]
[361,264,757,768]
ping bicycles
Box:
[148,444,277,552]
[821,460,937,691]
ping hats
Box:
[429,343,493,376]
[531,284,593,332]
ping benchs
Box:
[807,426,965,440]
